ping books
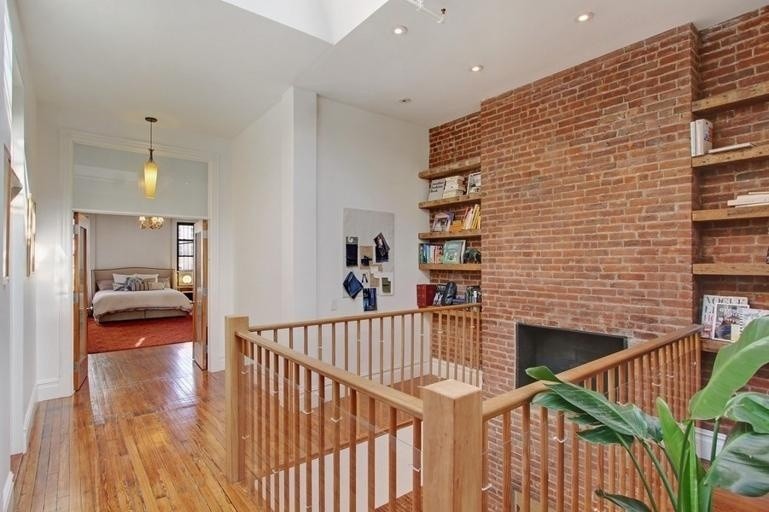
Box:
[691,190,768,345]
[417,166,482,313]
[708,142,755,153]
[696,118,713,156]
[690,120,697,158]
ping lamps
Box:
[142,116,159,200]
[138,216,165,231]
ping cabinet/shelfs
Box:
[419,158,483,315]
[688,86,769,357]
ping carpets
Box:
[86,314,195,353]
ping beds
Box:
[93,266,194,326]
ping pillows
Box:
[96,273,170,291]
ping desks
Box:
[177,285,193,302]
